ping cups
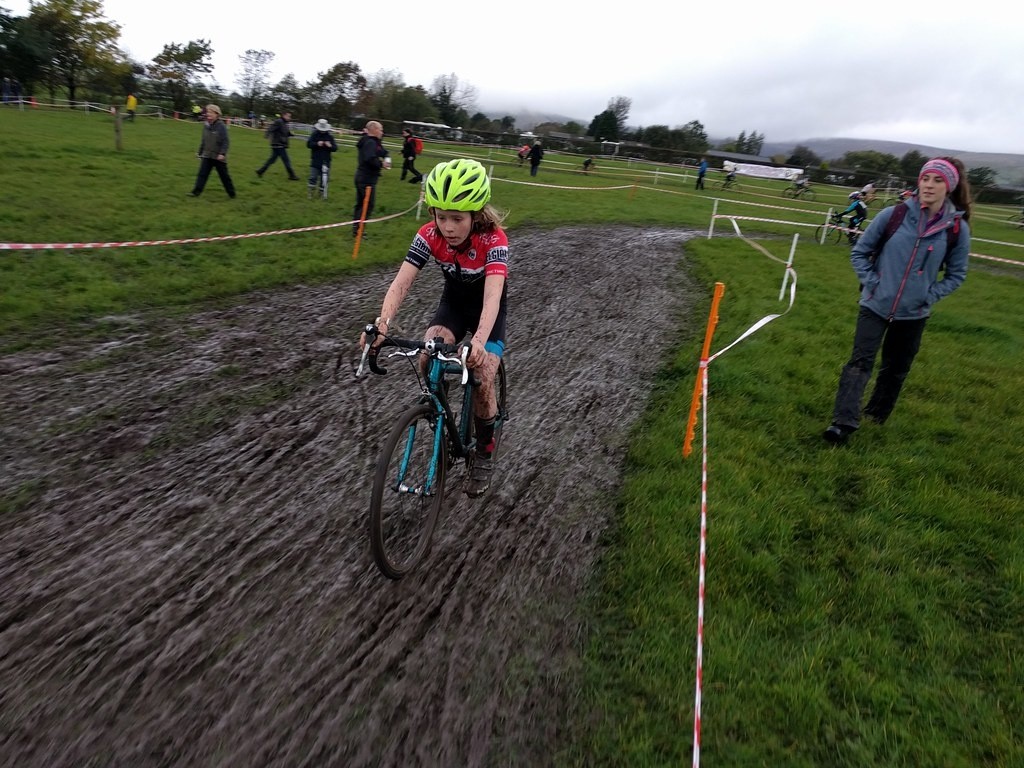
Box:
[385,157,391,170]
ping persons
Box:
[694,157,707,190]
[518,141,545,177]
[795,176,811,197]
[191,105,266,129]
[835,181,914,239]
[398,128,423,183]
[1,77,23,105]
[352,120,391,238]
[186,105,236,199]
[254,109,299,180]
[123,93,137,122]
[822,156,970,442]
[360,158,509,498]
[305,119,339,199]
[723,167,737,189]
[583,155,595,174]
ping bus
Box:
[402,120,455,143]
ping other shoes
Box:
[824,424,848,446]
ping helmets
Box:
[849,190,859,200]
[425,158,491,212]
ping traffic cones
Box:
[31,97,38,107]
[173,112,179,119]
[110,107,116,115]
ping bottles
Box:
[841,223,845,232]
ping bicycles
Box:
[815,207,868,246]
[509,156,532,168]
[575,164,599,178]
[357,317,508,579]
[884,197,905,207]
[712,177,740,193]
[847,196,884,209]
[1007,208,1024,233]
[782,183,815,201]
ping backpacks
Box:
[408,137,423,154]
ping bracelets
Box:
[375,316,392,329]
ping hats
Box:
[314,118,331,132]
[206,104,222,115]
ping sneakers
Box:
[467,453,494,499]
[430,380,448,430]
